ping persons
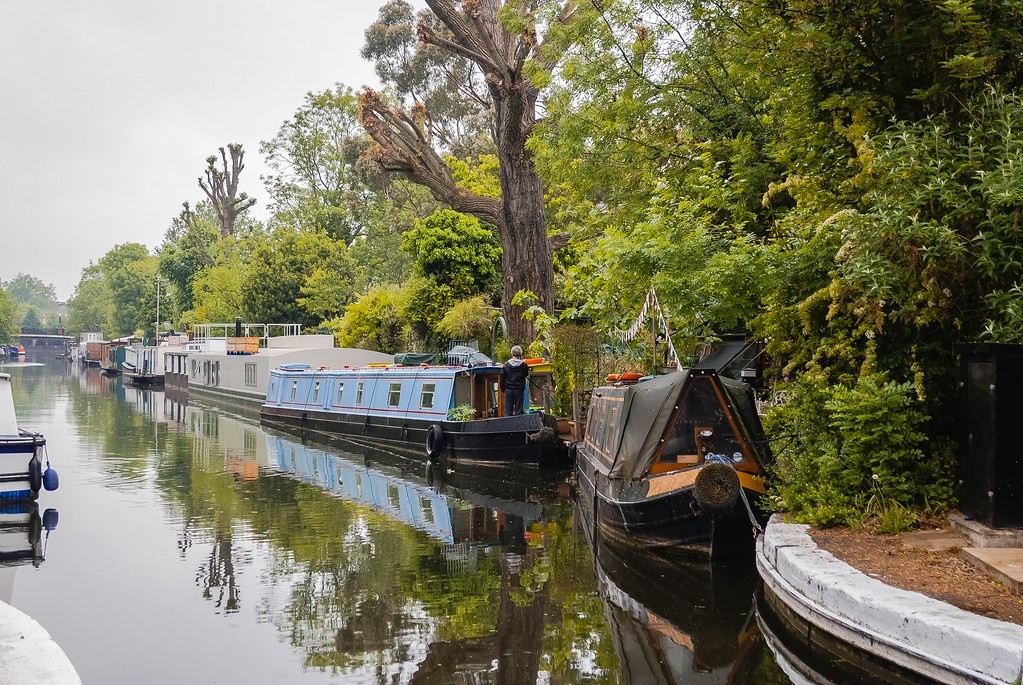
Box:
[500,346,529,417]
[498,512,526,588]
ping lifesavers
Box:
[425,424,443,458]
[523,357,546,364]
[608,373,644,380]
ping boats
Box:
[570,366,775,569]
[260,358,576,473]
[260,421,560,554]
[578,501,759,672]
[125,371,156,386]
[99,364,121,375]
